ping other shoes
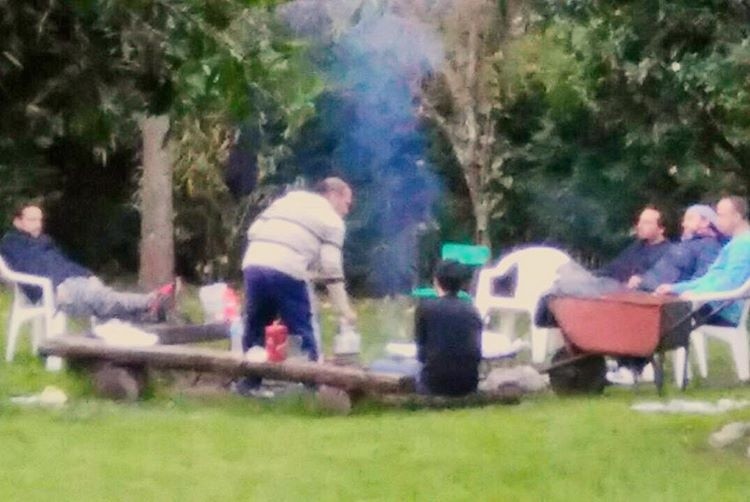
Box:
[605,367,634,385]
[637,363,655,383]
[149,277,182,321]
[236,379,275,399]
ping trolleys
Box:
[538,290,735,400]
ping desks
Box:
[534,291,693,397]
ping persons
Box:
[0,200,182,330]
[414,259,483,395]
[534,199,674,383]
[603,205,730,384]
[239,177,358,399]
[638,195,750,382]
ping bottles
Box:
[333,323,359,366]
[230,316,243,355]
[264,318,288,363]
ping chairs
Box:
[411,242,492,304]
[473,246,571,364]
[0,255,64,372]
[673,278,750,392]
